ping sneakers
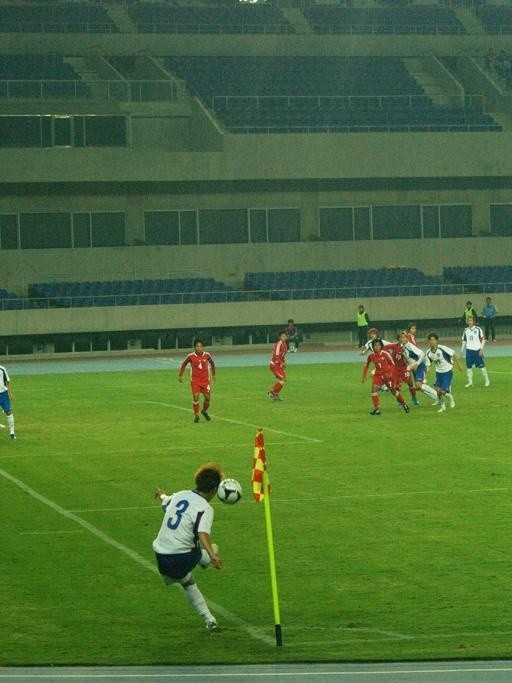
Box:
[201,544,219,569]
[369,381,490,415]
[201,411,210,420]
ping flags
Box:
[251,428,270,502]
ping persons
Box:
[284,296,498,416]
[153,461,223,630]
[490,45,511,92]
[1,362,17,441]
[266,332,287,403]
[176,339,216,425]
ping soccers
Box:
[218,479,242,503]
